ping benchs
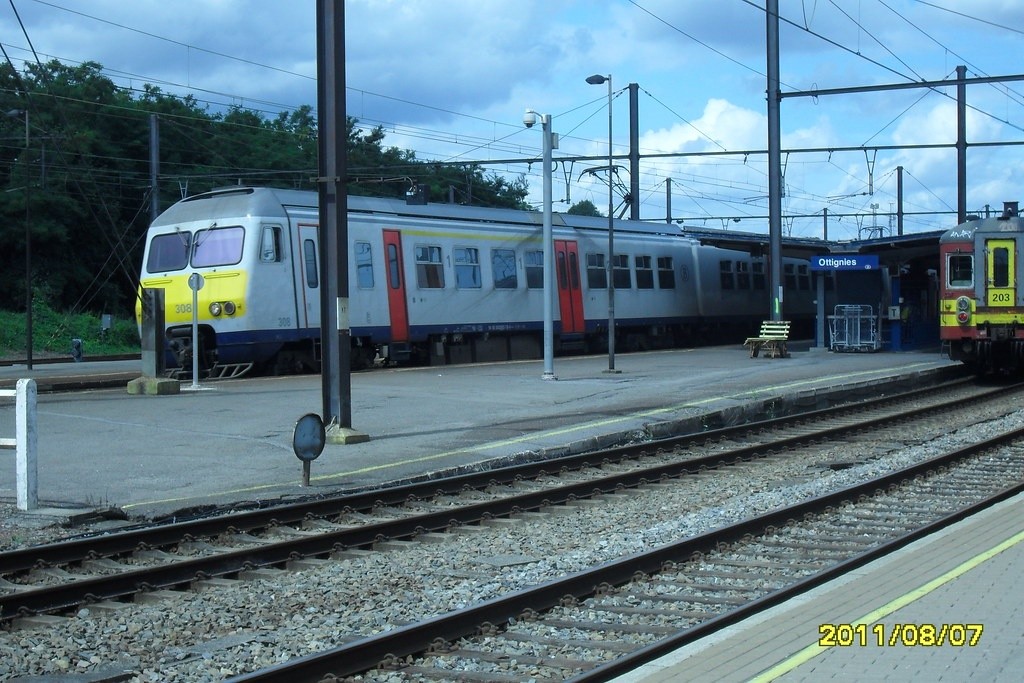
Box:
[744,320,791,359]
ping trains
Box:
[135,179,892,381]
[939,216,1024,382]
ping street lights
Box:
[586,73,618,372]
